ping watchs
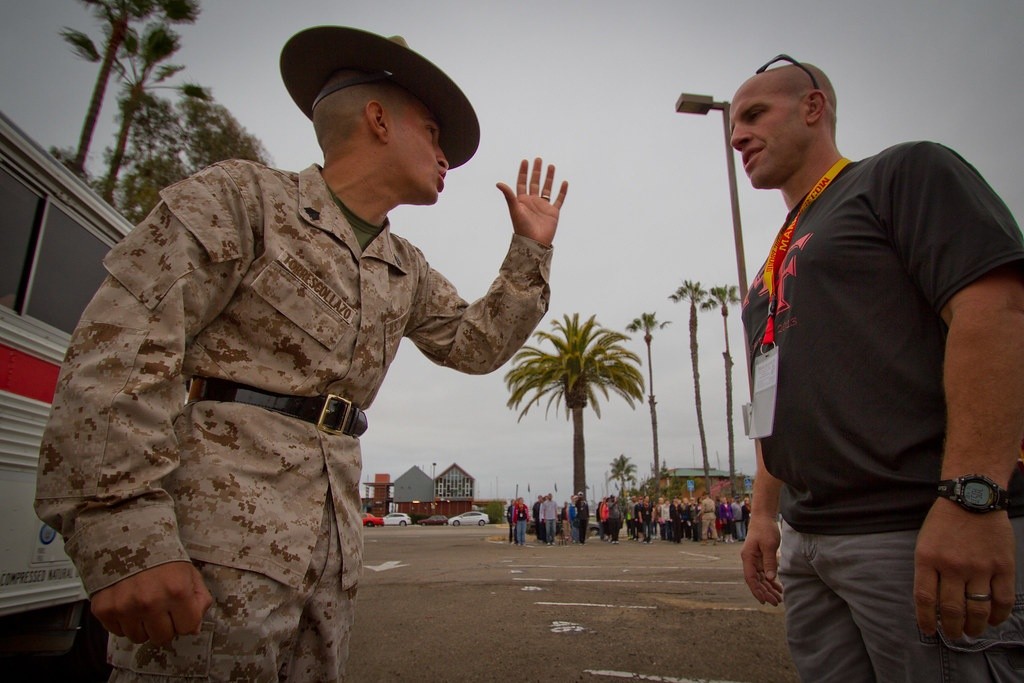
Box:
[936,473,1012,514]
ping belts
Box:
[704,510,714,513]
[184,375,368,438]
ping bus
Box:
[0,111,135,658]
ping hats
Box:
[578,492,583,495]
[280,25,480,170]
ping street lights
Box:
[675,93,754,402]
[432,463,437,498]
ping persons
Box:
[561,491,589,546]
[729,53,1024,683]
[595,494,624,544]
[628,491,751,545]
[507,497,531,547]
[533,493,558,546]
[33,25,569,683]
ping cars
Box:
[417,515,448,526]
[526,513,600,536]
[362,513,383,527]
[448,511,489,526]
[383,513,411,526]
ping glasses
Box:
[756,53,820,91]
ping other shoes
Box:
[547,542,551,546]
[612,540,619,543]
[649,541,653,544]
[725,538,728,543]
[552,542,556,546]
[642,541,647,544]
[729,539,734,543]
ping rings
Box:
[541,195,550,202]
[967,594,991,601]
